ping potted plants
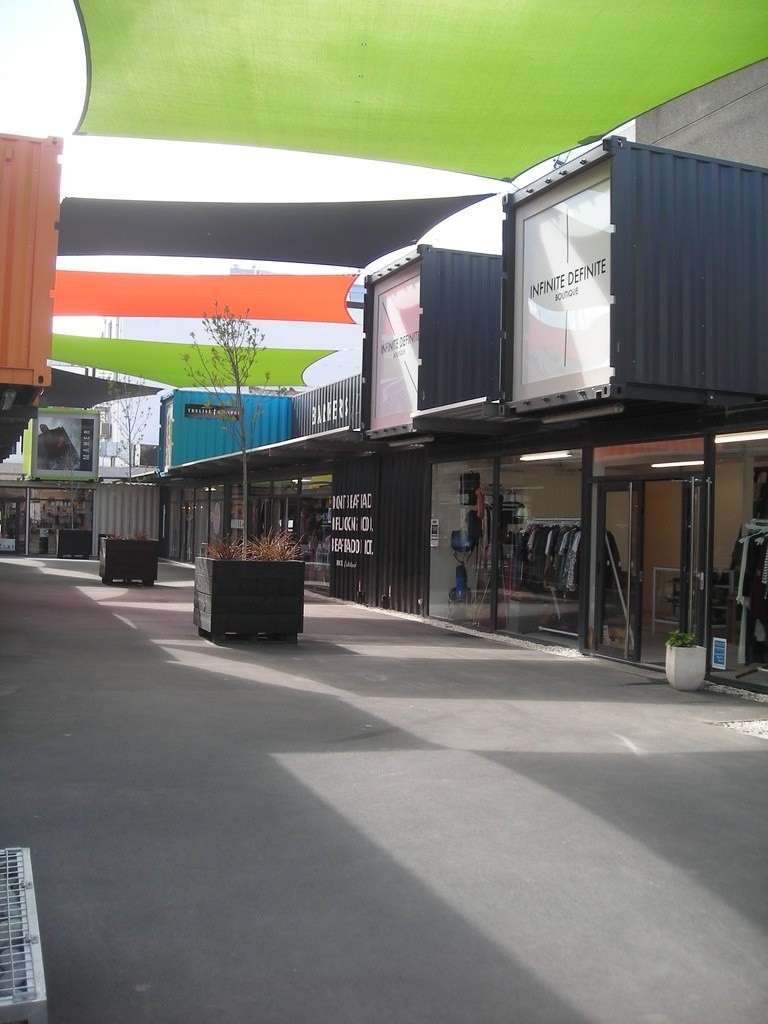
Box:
[98,370,158,585]
[665,629,707,691]
[0,510,20,554]
[179,301,305,644]
[54,440,93,559]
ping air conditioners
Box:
[99,422,111,436]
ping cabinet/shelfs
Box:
[652,568,733,637]
[457,504,514,595]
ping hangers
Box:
[530,517,581,527]
[739,519,768,546]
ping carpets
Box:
[649,661,735,672]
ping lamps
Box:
[652,460,704,468]
[715,429,768,444]
[520,450,573,461]
